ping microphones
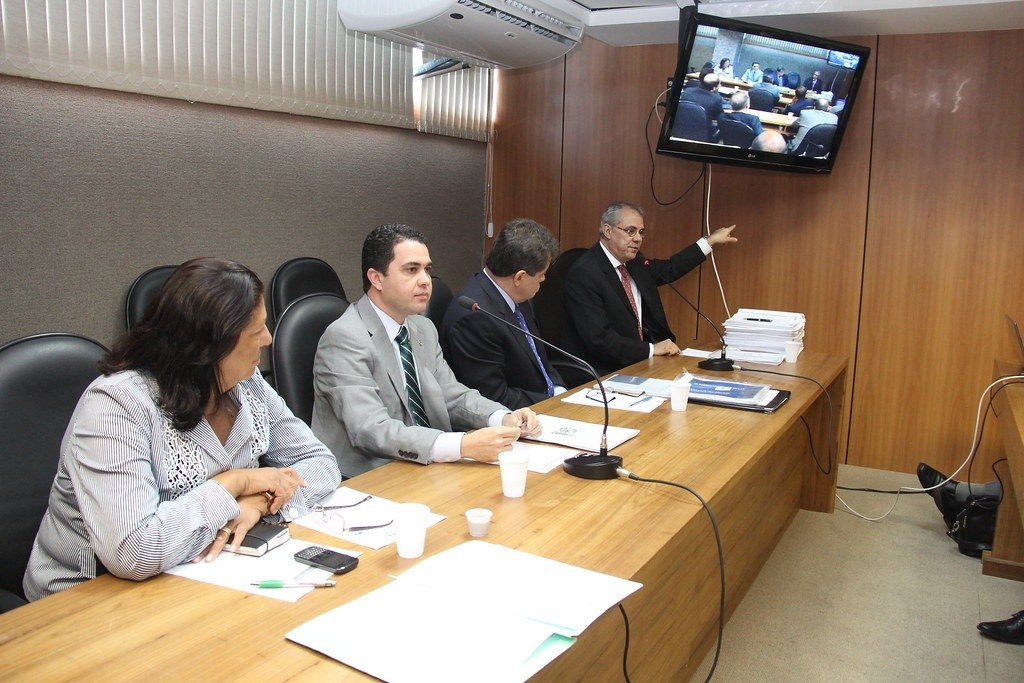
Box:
[458,296,623,480]
[638,253,735,371]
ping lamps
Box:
[337,0,585,71]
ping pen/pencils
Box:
[629,395,652,406]
[250,579,337,588]
[742,318,772,322]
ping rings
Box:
[221,527,231,535]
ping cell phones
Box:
[294,546,358,575]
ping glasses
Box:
[607,222,647,238]
[307,495,394,535]
[585,387,646,404]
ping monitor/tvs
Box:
[656,12,871,175]
[826,49,860,70]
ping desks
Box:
[686,72,802,136]
[981,360,1024,582]
[1,339,849,682]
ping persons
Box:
[681,73,724,119]
[917,463,1002,529]
[752,131,788,154]
[803,70,823,94]
[748,77,780,112]
[772,67,789,87]
[564,202,737,372]
[683,68,714,91]
[789,98,838,152]
[311,222,544,478]
[783,87,814,117]
[440,217,569,412]
[742,61,764,83]
[714,58,733,78]
[23,256,342,603]
[718,91,764,137]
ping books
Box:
[222,523,291,556]
[594,374,671,397]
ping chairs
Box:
[125,264,182,336]
[763,68,777,83]
[0,331,112,615]
[418,275,455,335]
[786,72,801,89]
[270,257,351,430]
[670,87,839,156]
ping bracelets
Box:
[704,236,712,248]
[258,491,274,501]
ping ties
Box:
[778,76,782,86]
[752,71,755,82]
[618,265,644,341]
[396,326,430,428]
[514,307,554,397]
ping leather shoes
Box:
[917,463,962,530]
[976,609,1024,644]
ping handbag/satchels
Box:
[946,495,999,556]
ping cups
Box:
[784,341,802,363]
[788,112,794,119]
[670,381,692,412]
[392,503,430,559]
[734,86,739,91]
[734,77,739,82]
[465,508,493,538]
[498,451,530,498]
